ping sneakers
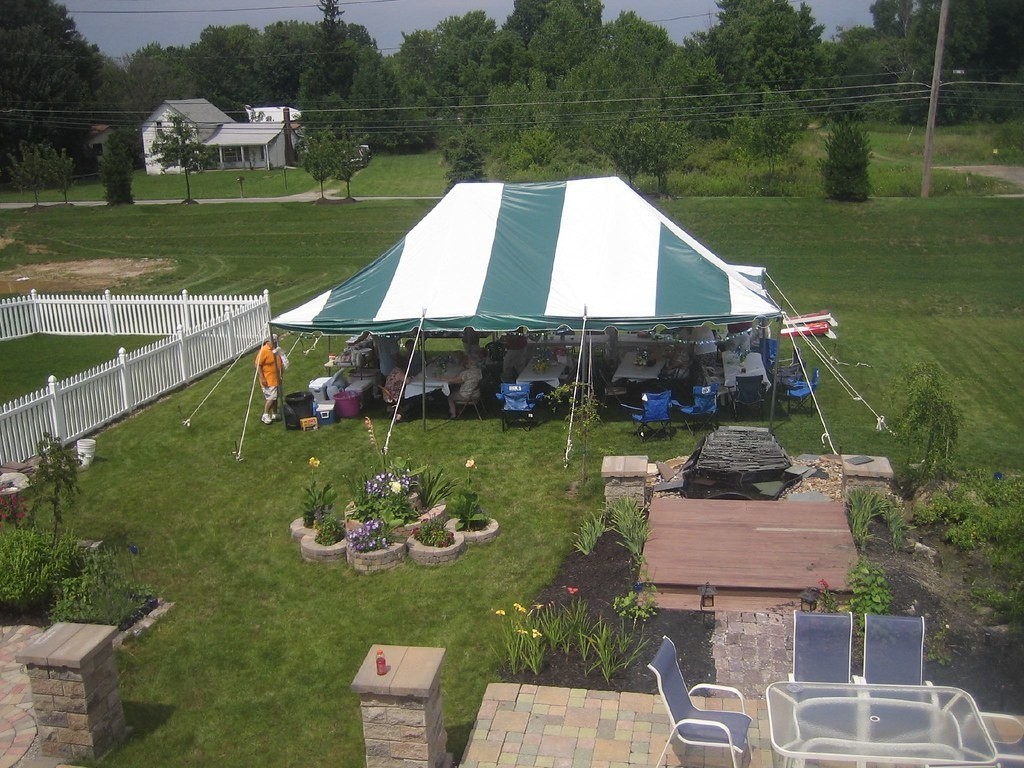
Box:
[262,414,272,425]
[272,414,281,419]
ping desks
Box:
[515,355,573,408]
[613,352,667,395]
[722,350,771,401]
[766,681,998,768]
[497,334,677,361]
[380,328,497,353]
[323,350,379,379]
[406,354,470,414]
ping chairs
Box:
[860,613,940,768]
[382,325,820,443]
[787,610,860,768]
[647,634,753,768]
[954,712,1024,768]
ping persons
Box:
[500,327,528,385]
[751,319,770,352]
[719,321,749,354]
[354,332,418,420]
[255,334,283,424]
[434,358,481,419]
[659,325,718,395]
[461,328,479,357]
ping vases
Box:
[440,363,446,372]
[503,348,522,375]
[536,365,544,373]
[739,357,745,367]
[561,334,565,340]
[638,358,647,366]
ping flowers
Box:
[491,603,544,675]
[487,342,505,362]
[433,354,452,374]
[122,544,146,597]
[817,578,843,614]
[732,345,750,357]
[300,417,486,552]
[614,575,657,620]
[500,332,527,350]
[0,482,33,529]
[634,345,650,366]
[533,347,552,373]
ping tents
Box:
[267,177,785,434]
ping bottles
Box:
[375,650,387,675]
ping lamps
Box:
[698,583,718,614]
[801,587,819,613]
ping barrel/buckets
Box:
[77,438,96,464]
[334,392,360,418]
[284,392,313,430]
